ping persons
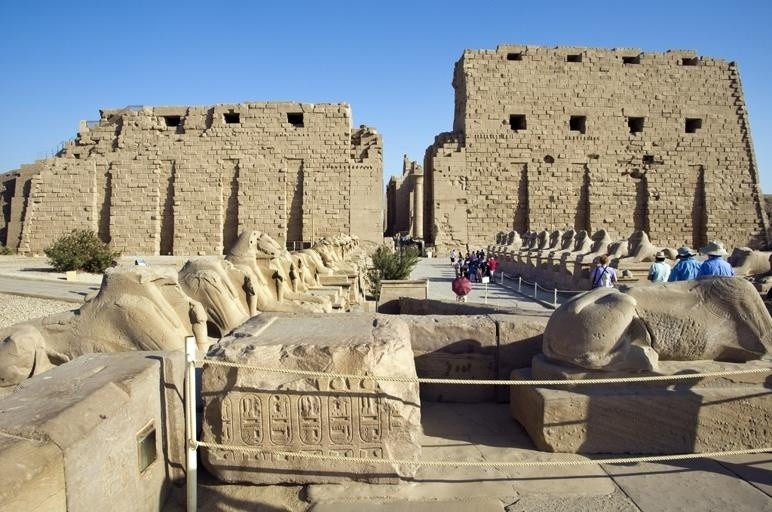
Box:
[668,247,701,282]
[449,249,497,301]
[697,240,736,280]
[648,252,672,283]
[400,237,414,247]
[590,255,617,288]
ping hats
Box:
[675,246,696,259]
[653,251,669,258]
[700,241,729,256]
